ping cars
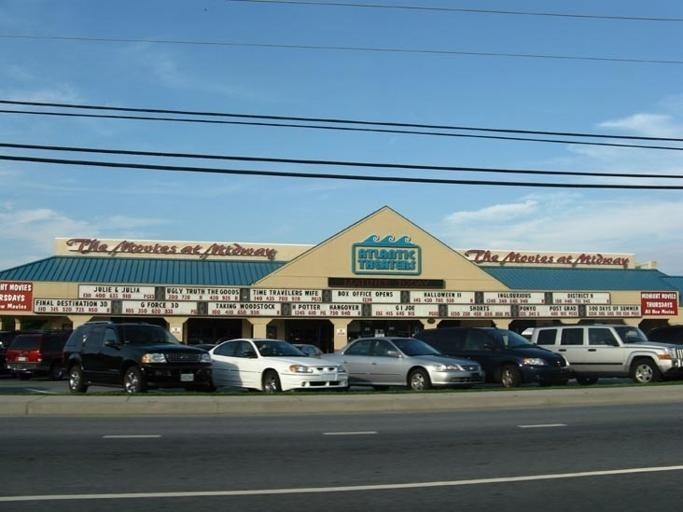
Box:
[309,336,483,391]
[0,331,50,378]
[6,329,73,381]
[209,337,349,396]
[292,344,323,357]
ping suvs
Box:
[529,323,682,386]
[62,321,213,395]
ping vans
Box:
[411,325,570,388]
